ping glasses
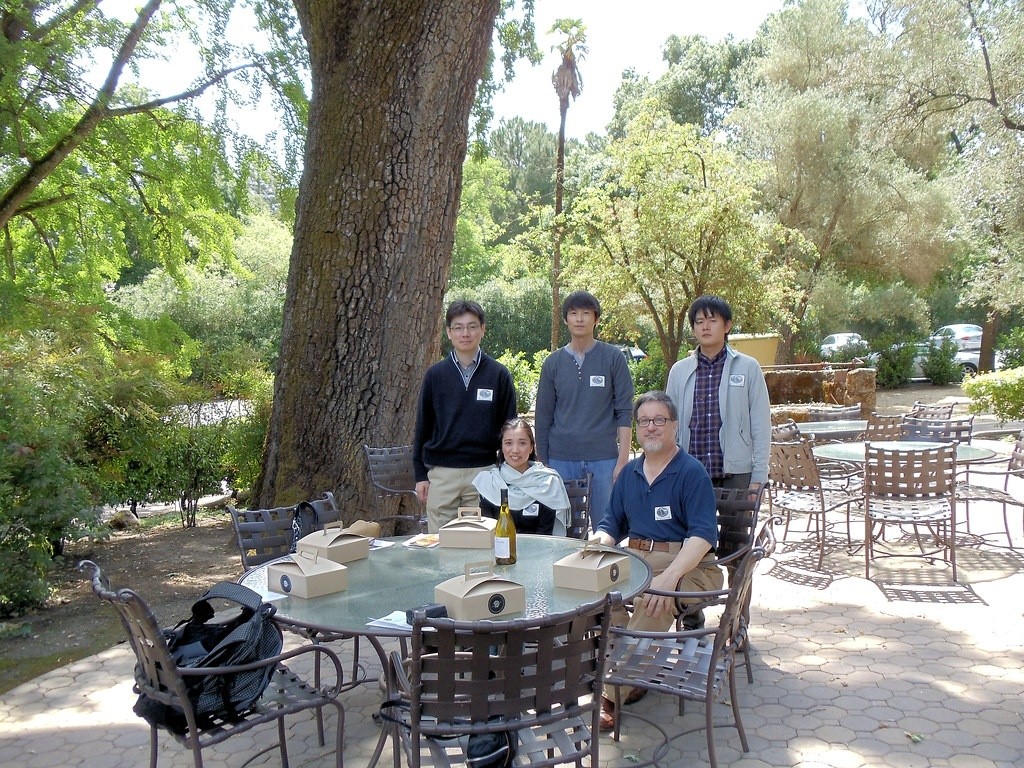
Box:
[636,417,674,428]
[449,325,481,333]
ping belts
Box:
[629,539,715,553]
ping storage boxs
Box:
[433,560,527,619]
[265,551,349,597]
[296,521,368,563]
[551,537,633,594]
[440,506,498,548]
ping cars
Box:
[813,323,985,384]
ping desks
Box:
[795,422,899,492]
[239,536,652,767]
[812,439,996,557]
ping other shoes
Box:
[488,671,495,679]
[676,625,707,647]
[731,635,750,652]
[408,645,438,657]
[623,687,648,705]
[592,694,614,730]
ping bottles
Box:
[494,488,517,564]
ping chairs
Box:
[75,402,1024,767]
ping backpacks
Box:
[133,581,283,734]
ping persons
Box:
[666,293,772,651]
[593,390,723,731]
[412,301,516,535]
[535,290,633,551]
[471,419,571,680]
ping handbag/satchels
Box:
[289,501,327,553]
[465,715,518,768]
